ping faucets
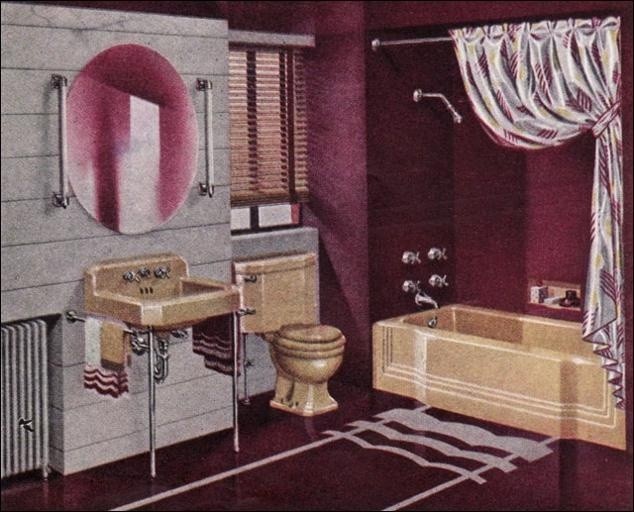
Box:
[414,289,440,309]
[138,267,151,280]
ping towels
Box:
[83,317,132,401]
[193,312,242,377]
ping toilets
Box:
[232,251,346,418]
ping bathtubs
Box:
[371,303,627,452]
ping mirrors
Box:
[65,44,199,237]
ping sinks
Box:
[83,253,241,328]
